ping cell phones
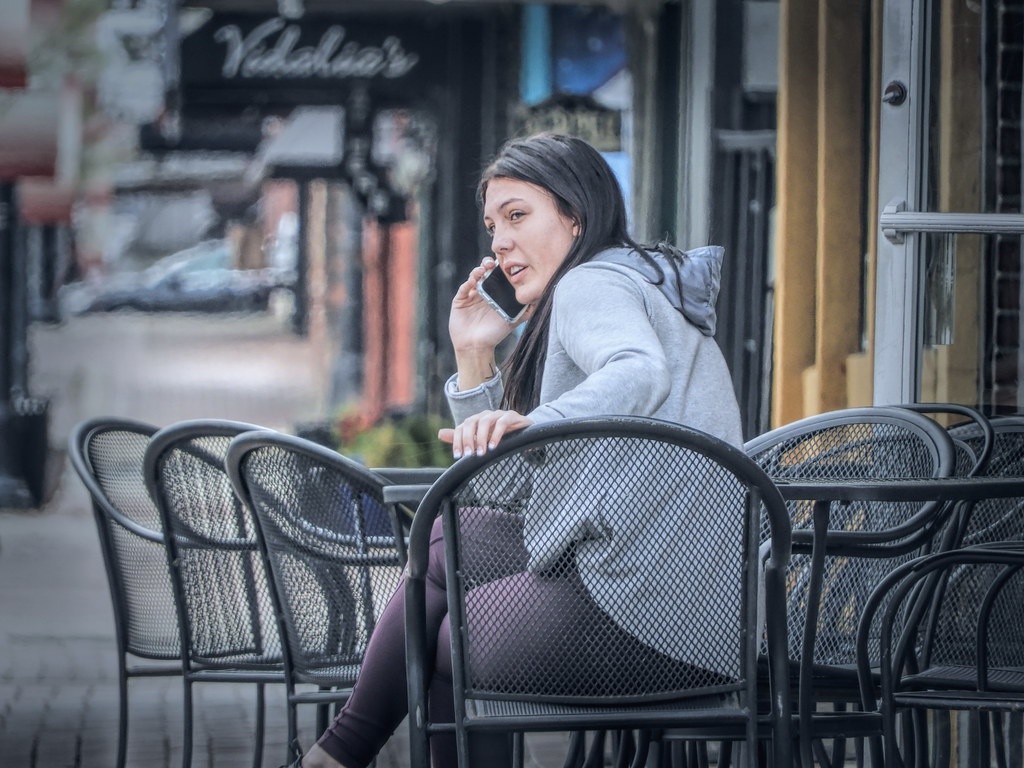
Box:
[476,259,530,324]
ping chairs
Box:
[66,404,1023,768]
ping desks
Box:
[382,480,1024,768]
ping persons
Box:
[280,134,747,768]
